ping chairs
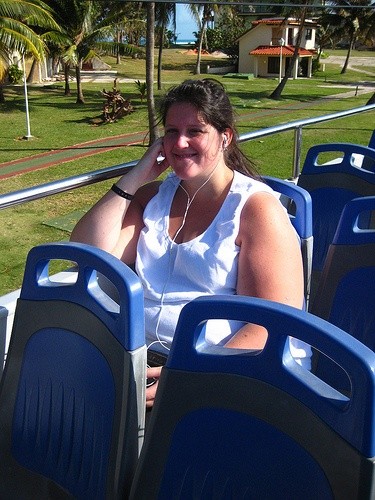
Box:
[129,296,375,500]
[249,130,375,352]
[0,242,147,500]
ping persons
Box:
[68,77,305,423]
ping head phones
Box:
[224,135,228,149]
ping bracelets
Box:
[110,183,135,201]
